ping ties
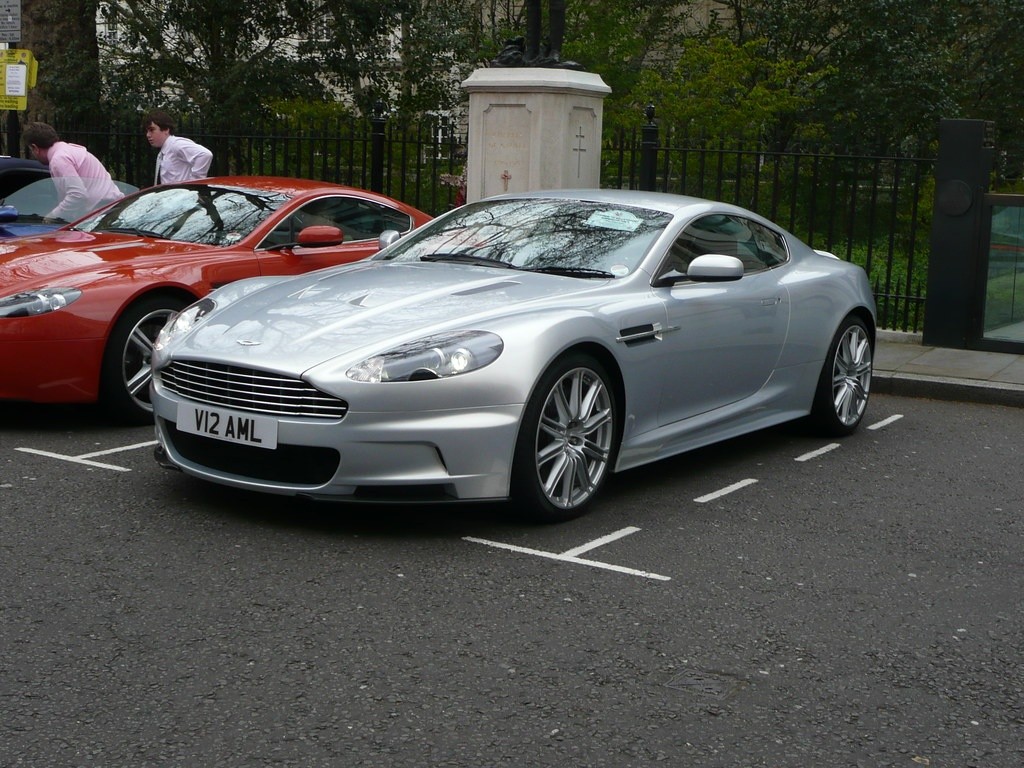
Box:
[157,153,163,185]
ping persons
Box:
[145,112,213,189]
[23,122,125,225]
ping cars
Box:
[149,189,880,520]
[0,153,436,431]
